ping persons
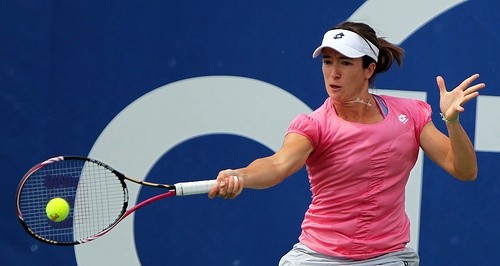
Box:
[208,22,486,266]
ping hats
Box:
[312,29,379,62]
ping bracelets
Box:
[439,112,459,123]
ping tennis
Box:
[45,197,71,224]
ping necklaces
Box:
[344,94,373,108]
